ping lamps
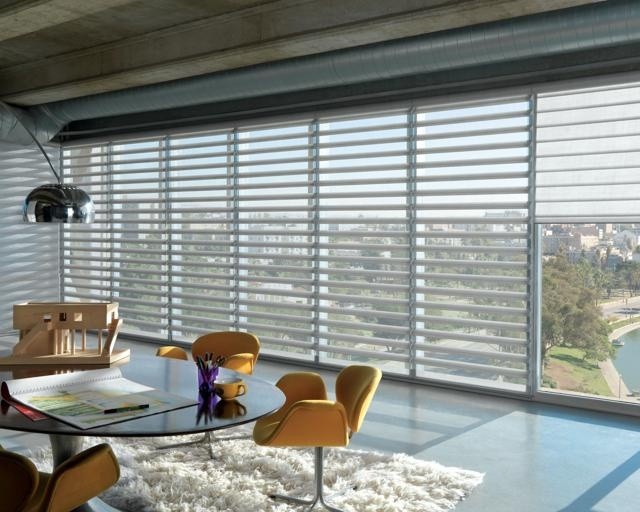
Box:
[0,99,96,224]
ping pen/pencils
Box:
[196,352,225,391]
[104,404,149,413]
[196,392,216,427]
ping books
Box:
[0,366,202,431]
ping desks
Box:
[0,353,287,469]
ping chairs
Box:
[1,437,121,511]
[155,331,261,376]
[255,364,384,511]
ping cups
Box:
[198,368,247,401]
[196,397,248,420]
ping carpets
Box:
[12,425,487,511]
